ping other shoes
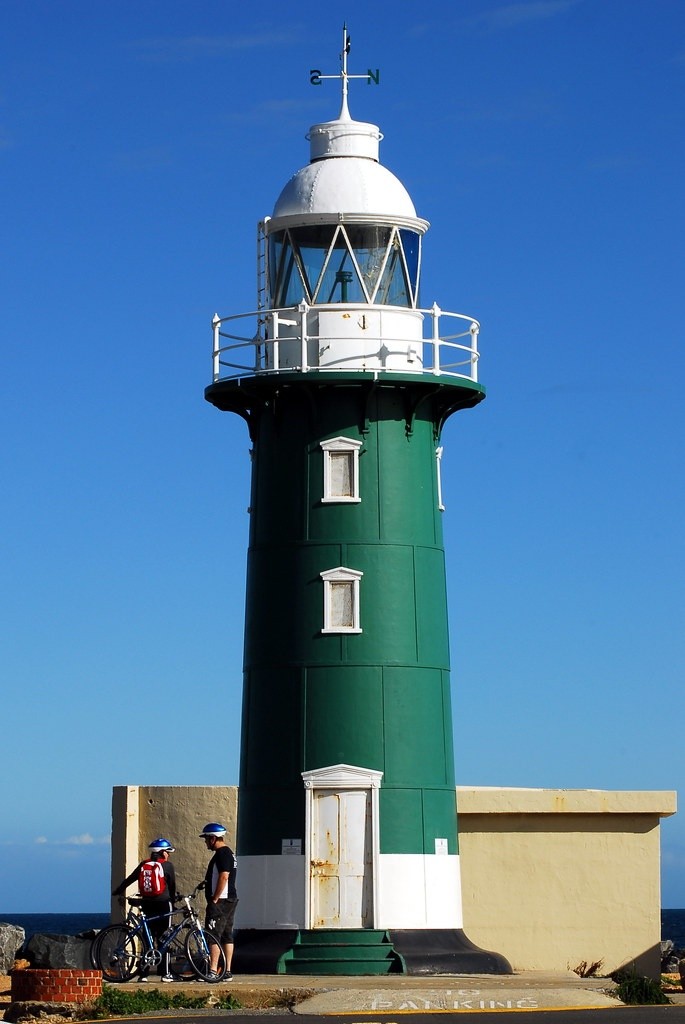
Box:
[161,972,183,982]
[222,971,234,981]
[137,976,148,982]
[197,970,217,982]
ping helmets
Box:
[148,838,175,853]
[199,823,226,839]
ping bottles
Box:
[159,926,174,943]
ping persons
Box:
[196,823,239,982]
[111,839,186,983]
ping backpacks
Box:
[139,858,166,896]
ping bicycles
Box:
[89,881,227,984]
